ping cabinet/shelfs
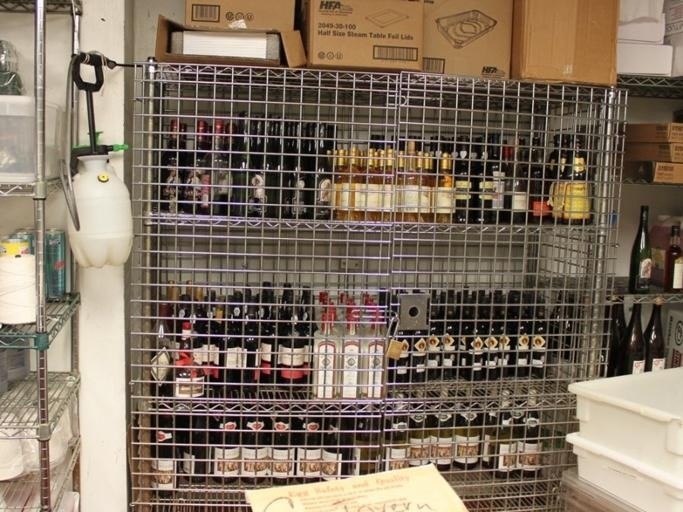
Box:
[0,0,82,512]
[141,56,682,512]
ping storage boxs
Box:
[423,0,513,79]
[154,13,308,68]
[304,0,424,72]
[512,0,619,87]
[554,367,682,511]
[185,0,295,32]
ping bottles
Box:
[149,116,609,224]
[152,275,607,396]
[145,390,558,492]
[626,205,653,293]
[665,225,683,293]
[620,296,649,377]
[642,298,666,372]
[606,294,625,378]
